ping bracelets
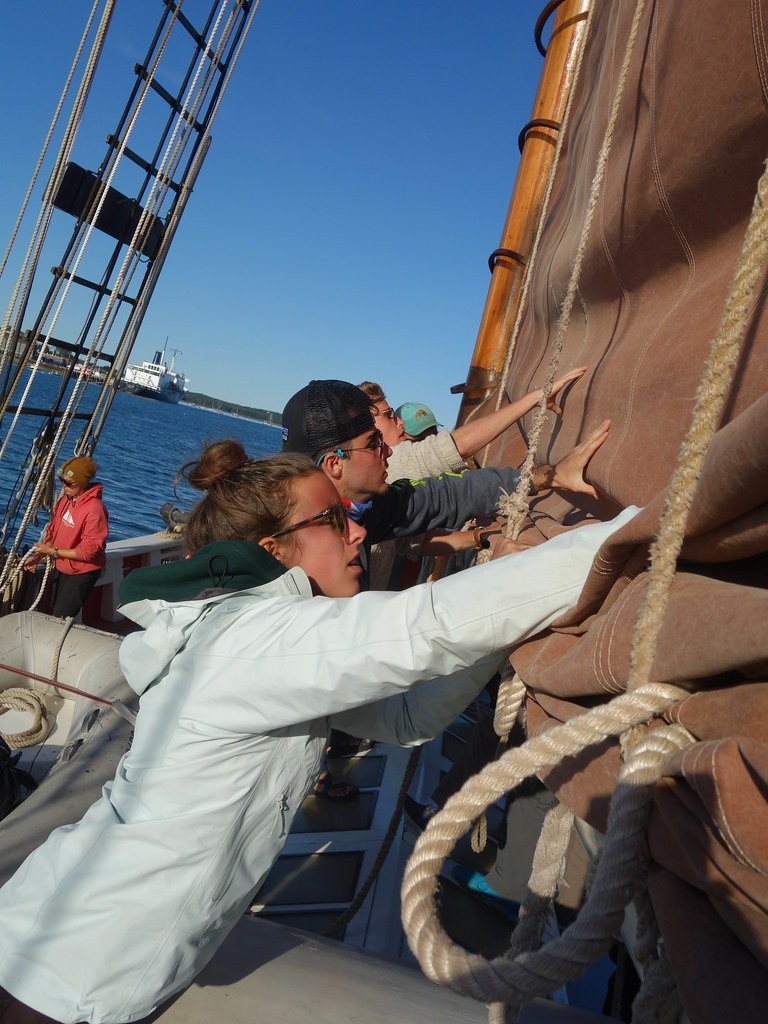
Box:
[53,548,59,558]
[474,526,486,546]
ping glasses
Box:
[375,408,395,420]
[270,506,348,537]
[316,433,384,467]
[58,475,76,487]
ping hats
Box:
[60,457,96,488]
[395,402,444,436]
[280,380,373,457]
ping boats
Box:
[124,334,190,404]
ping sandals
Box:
[311,772,359,801]
[325,738,372,758]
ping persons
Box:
[0,441,643,1024]
[395,402,444,443]
[311,367,589,799]
[281,378,610,590]
[24,457,109,620]
